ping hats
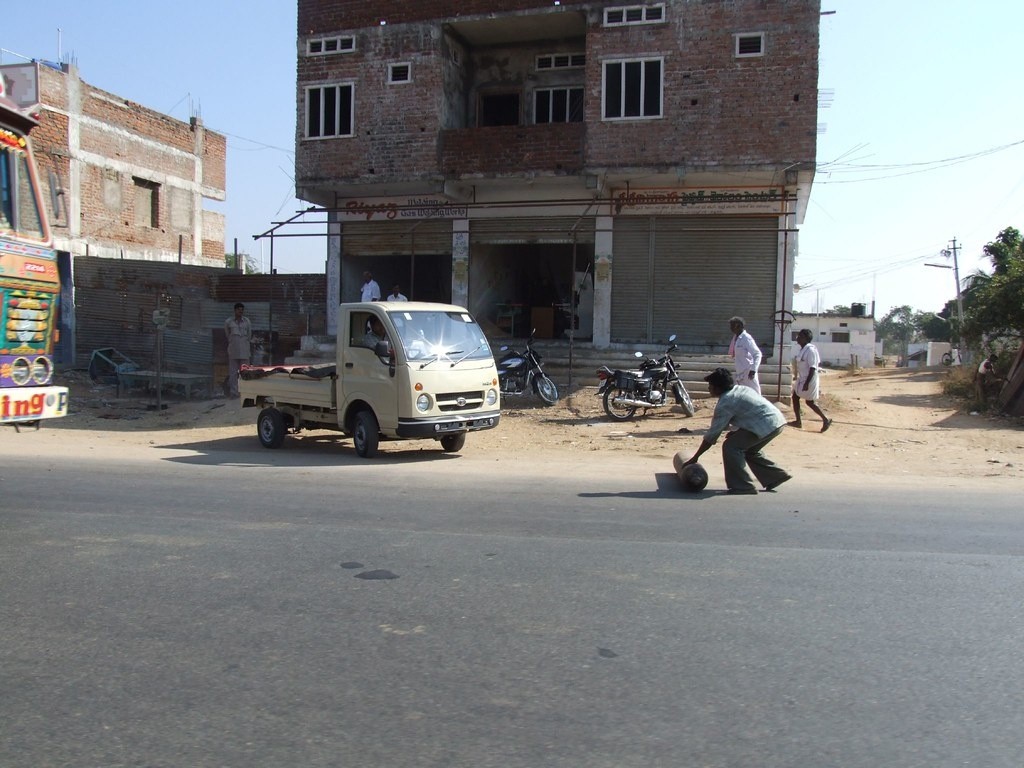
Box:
[705,367,735,391]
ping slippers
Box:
[788,421,802,429]
[821,418,832,432]
[766,475,793,491]
[727,486,758,495]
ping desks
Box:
[530,306,571,340]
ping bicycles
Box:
[941,351,961,366]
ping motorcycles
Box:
[595,334,696,421]
[498,328,559,406]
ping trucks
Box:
[236,300,501,457]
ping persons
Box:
[363,315,395,364]
[728,316,761,395]
[680,367,792,495]
[787,328,833,433]
[360,272,380,302]
[388,284,407,336]
[976,354,997,405]
[225,303,251,399]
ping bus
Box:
[1,99,74,427]
